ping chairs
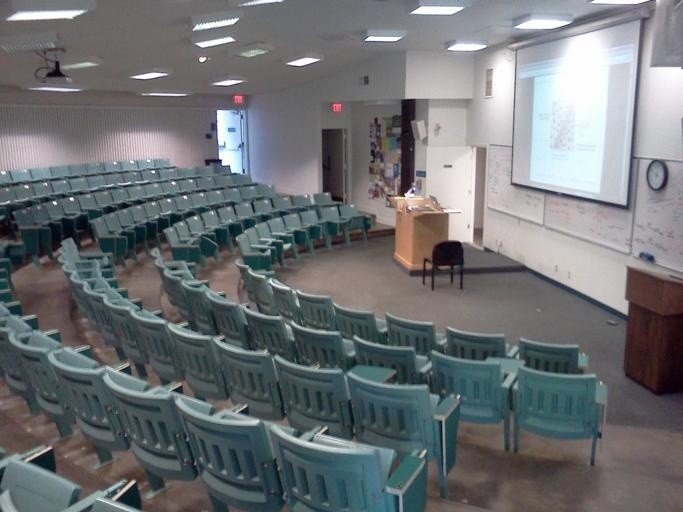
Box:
[422,240,463,291]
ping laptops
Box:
[428,194,453,210]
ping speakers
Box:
[411,119,427,140]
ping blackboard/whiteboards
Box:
[545,157,638,255]
[631,156,683,274]
[485,144,544,226]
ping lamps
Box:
[211,75,247,86]
[285,52,322,67]
[1,2,91,22]
[188,12,241,33]
[61,55,103,70]
[408,1,467,15]
[444,38,487,52]
[362,28,405,43]
[512,12,572,30]
[234,41,273,59]
[128,72,168,80]
[136,88,191,97]
[404,181,418,214]
[193,35,235,49]
[27,46,82,93]
[587,0,651,5]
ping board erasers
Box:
[639,252,655,263]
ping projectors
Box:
[38,60,74,86]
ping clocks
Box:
[646,159,667,191]
